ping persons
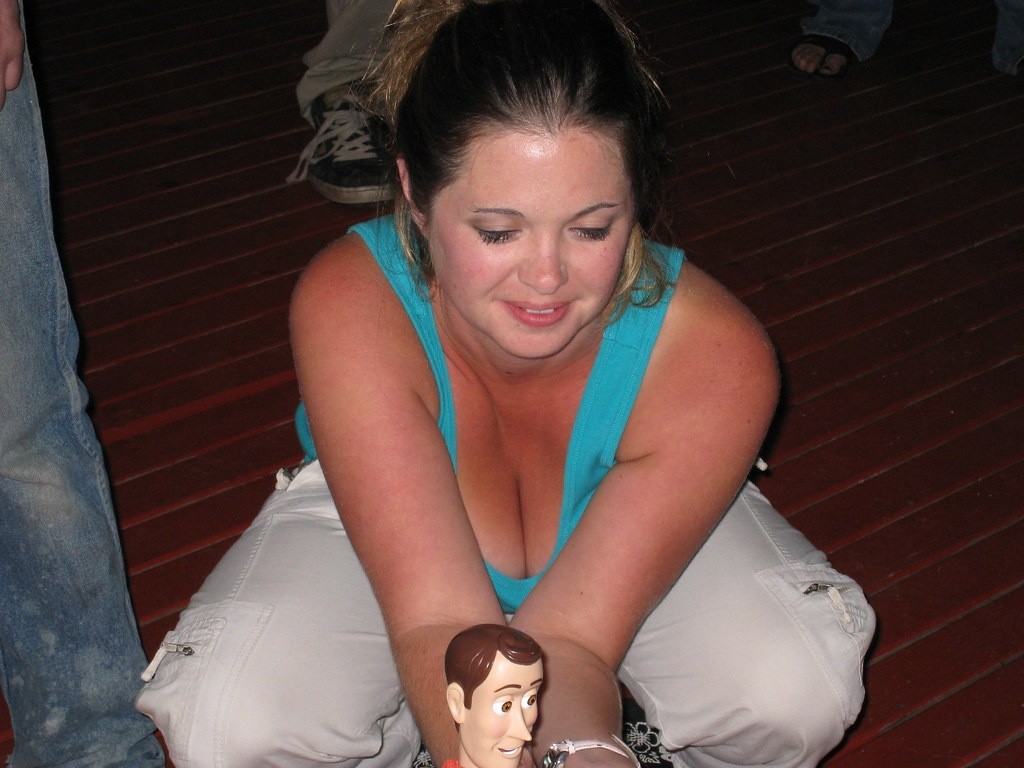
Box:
[0,2,167,768]
[441,623,543,768]
[284,0,403,205]
[785,0,1024,85]
[134,0,876,768]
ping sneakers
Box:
[286,75,399,204]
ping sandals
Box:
[785,34,853,81]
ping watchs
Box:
[539,732,641,768]
